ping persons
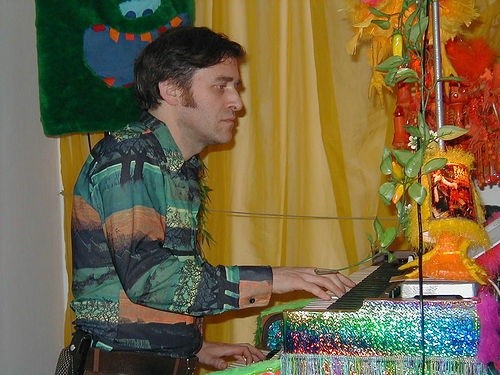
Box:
[51,23,358,375]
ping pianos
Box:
[205,218,500,375]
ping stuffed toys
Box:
[29,0,196,139]
[390,143,492,290]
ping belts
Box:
[82,348,199,375]
